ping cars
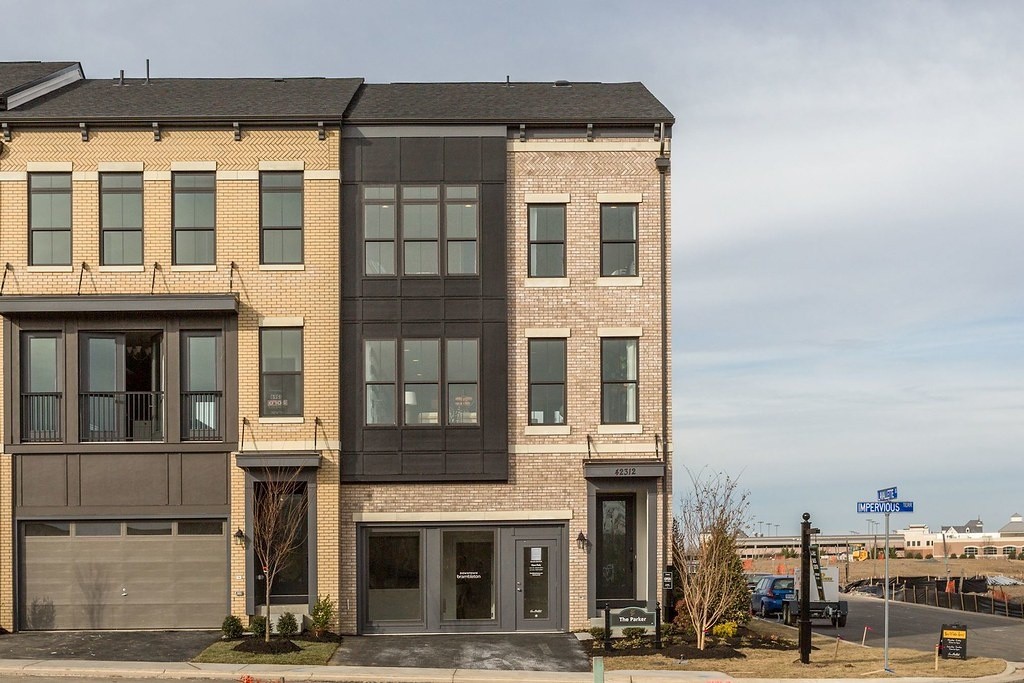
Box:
[750,575,794,618]
[742,573,773,590]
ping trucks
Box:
[782,544,848,627]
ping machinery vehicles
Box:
[849,544,868,562]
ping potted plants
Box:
[310,593,336,639]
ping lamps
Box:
[576,530,587,552]
[127,337,152,361]
[405,391,418,424]
[233,528,244,547]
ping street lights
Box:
[871,521,875,534]
[774,525,780,536]
[866,519,872,535]
[798,513,820,664]
[767,523,772,535]
[874,523,880,534]
[757,521,764,533]
